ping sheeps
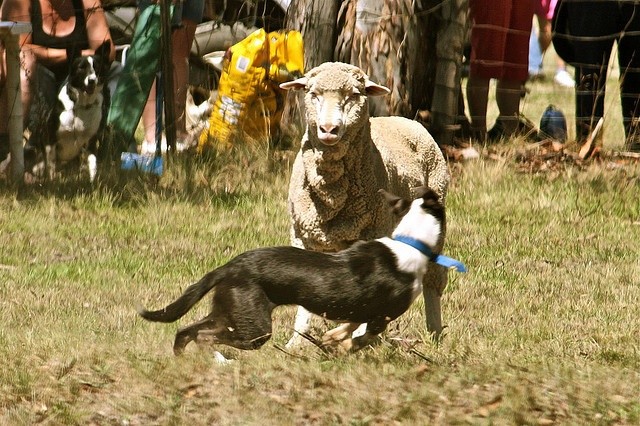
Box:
[280,62,450,350]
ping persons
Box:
[138,0,206,155]
[574,1,640,153]
[0,0,115,140]
[466,0,557,148]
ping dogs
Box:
[135,186,468,365]
[24,39,112,188]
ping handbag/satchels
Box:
[540,104,568,143]
